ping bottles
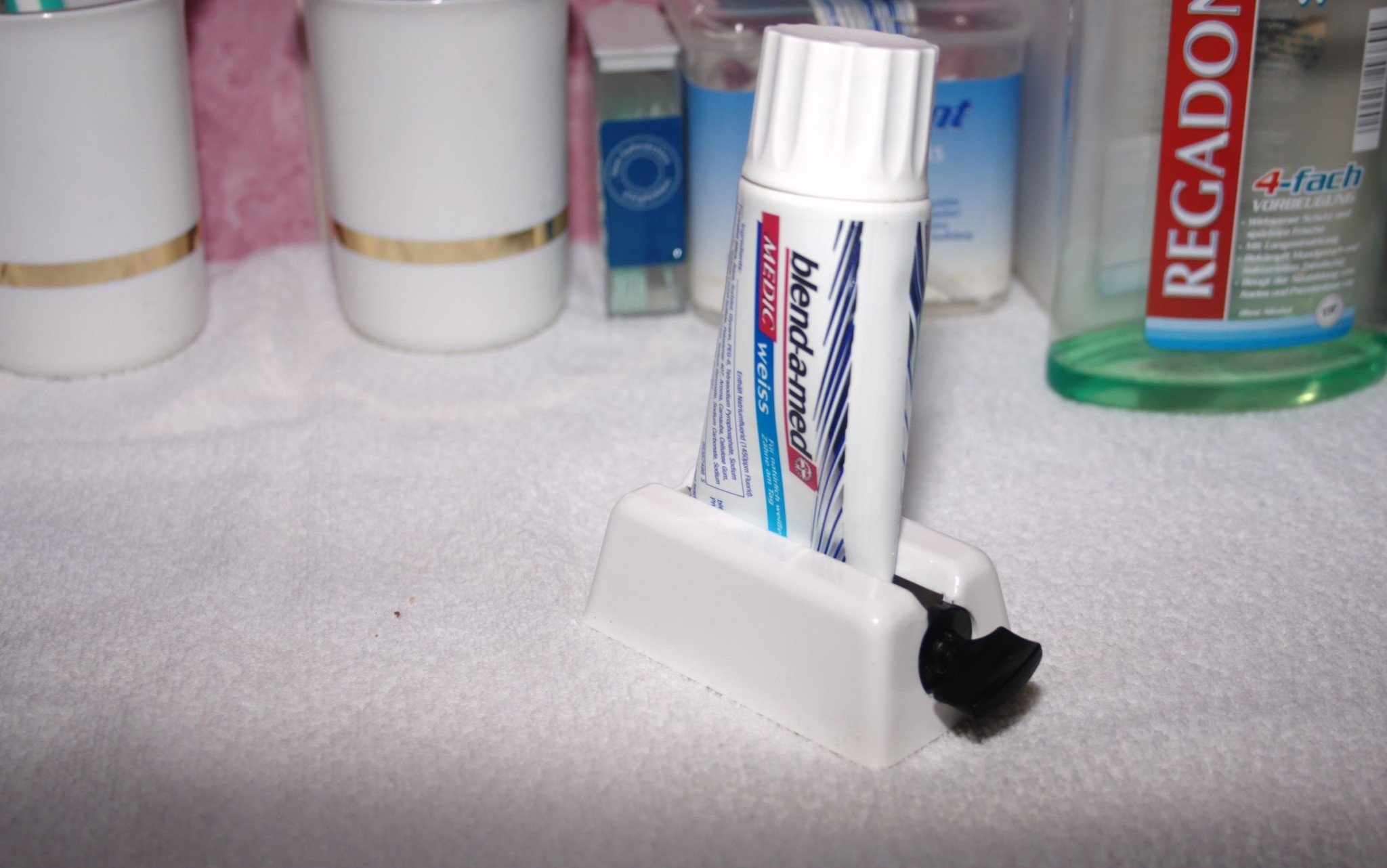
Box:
[1045,0,1387,413]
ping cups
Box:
[0,0,209,377]
[301,0,568,349]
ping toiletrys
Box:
[692,16,946,582]
